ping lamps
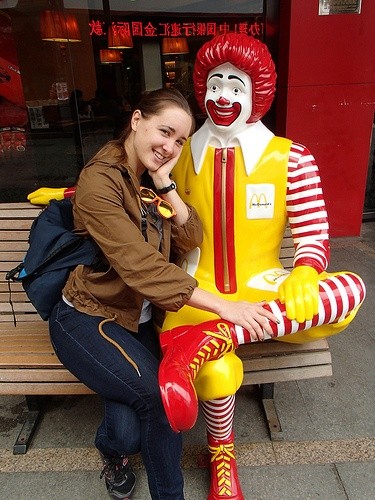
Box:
[39,10,189,172]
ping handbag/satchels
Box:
[4,195,105,321]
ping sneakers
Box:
[97,450,136,499]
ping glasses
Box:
[138,187,177,219]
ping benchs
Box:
[0,202,333,454]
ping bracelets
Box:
[158,181,177,193]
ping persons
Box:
[48,87,281,500]
[159,32,366,500]
[67,86,113,108]
